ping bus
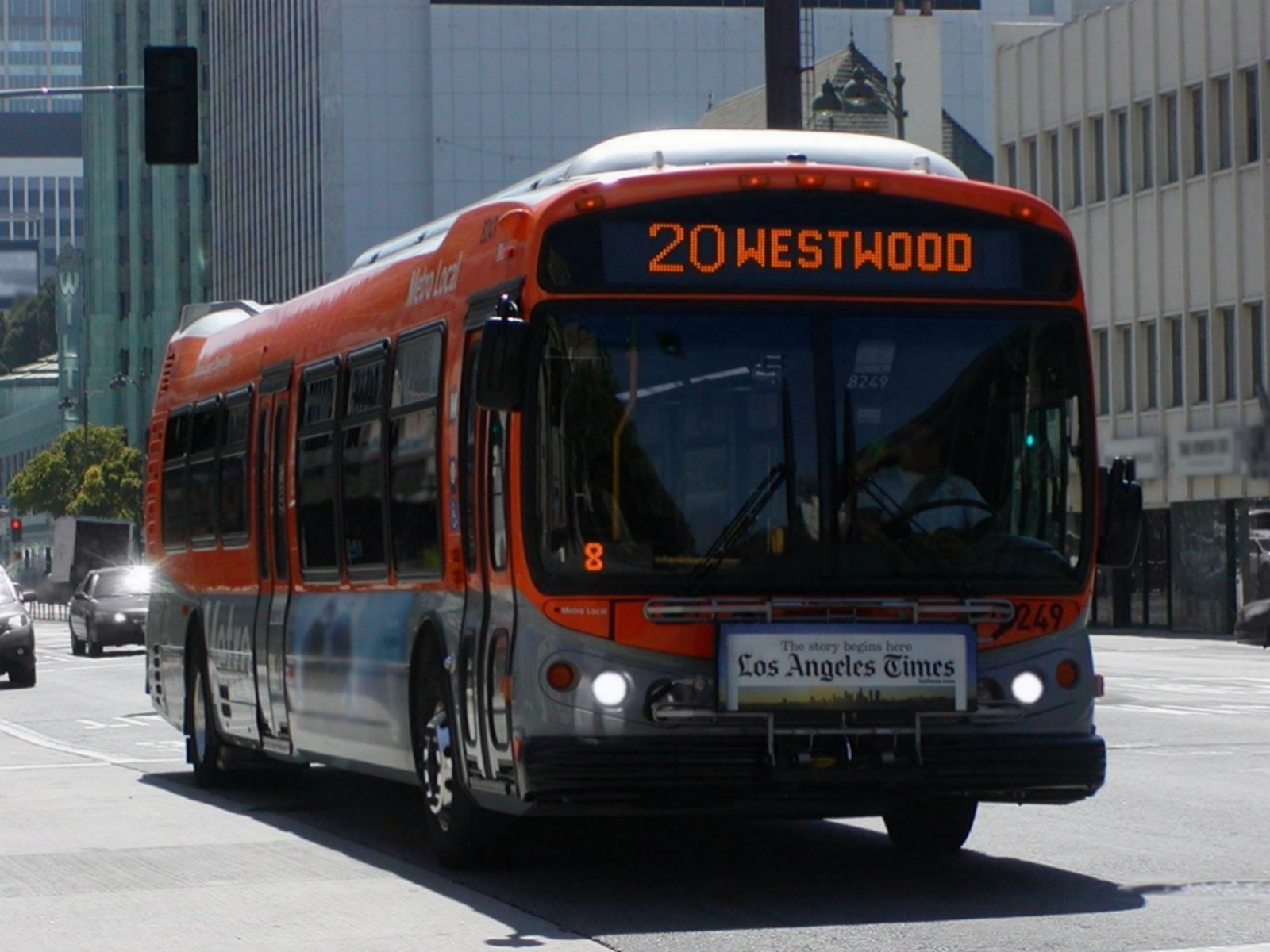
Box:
[147,128,1142,873]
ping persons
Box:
[856,410,997,555]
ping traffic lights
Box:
[144,46,198,166]
[11,520,22,541]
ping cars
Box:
[0,565,37,688]
[1235,598,1270,649]
[69,567,151,658]
[1248,536,1270,575]
[1248,508,1269,542]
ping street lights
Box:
[813,61,909,140]
[59,392,89,469]
